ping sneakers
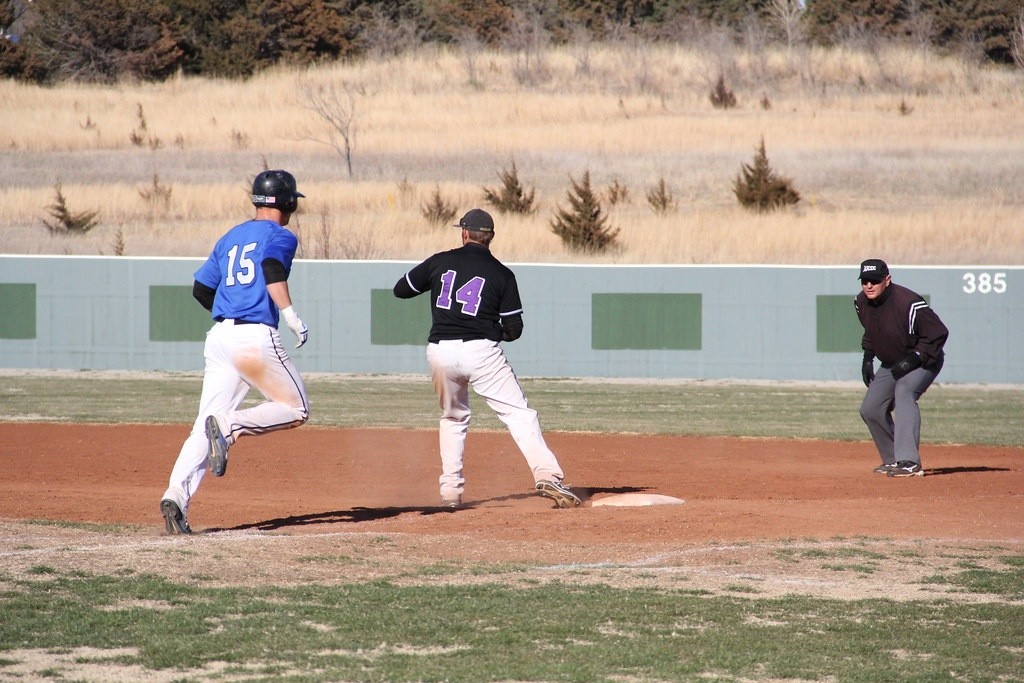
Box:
[535,479,582,508]
[440,499,461,508]
[887,460,924,476]
[873,462,898,474]
[160,499,192,535]
[205,414,235,478]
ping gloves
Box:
[281,305,308,348]
[890,352,922,380]
[862,360,875,388]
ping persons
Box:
[394,209,581,510]
[854,259,949,478]
[159,170,310,535]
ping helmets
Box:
[251,169,305,215]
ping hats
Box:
[453,209,494,232]
[857,259,889,280]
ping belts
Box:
[430,338,484,344]
[216,317,260,325]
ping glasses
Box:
[861,278,883,285]
